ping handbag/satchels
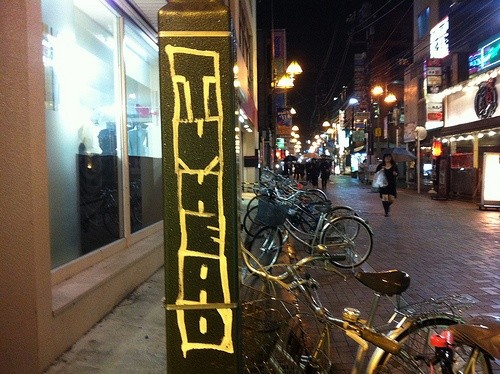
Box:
[372,168,388,189]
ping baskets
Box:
[253,169,290,195]
[240,283,331,374]
[257,194,289,228]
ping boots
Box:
[382,200,392,217]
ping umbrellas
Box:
[303,152,320,159]
[376,148,416,165]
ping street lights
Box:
[371,84,397,150]
[271,54,303,172]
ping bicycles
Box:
[236,227,495,373]
[240,166,376,277]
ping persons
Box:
[283,162,290,175]
[317,155,333,180]
[289,161,293,175]
[319,158,331,191]
[293,163,305,179]
[308,158,321,189]
[305,160,313,183]
[375,154,399,218]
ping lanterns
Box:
[432,142,442,157]
